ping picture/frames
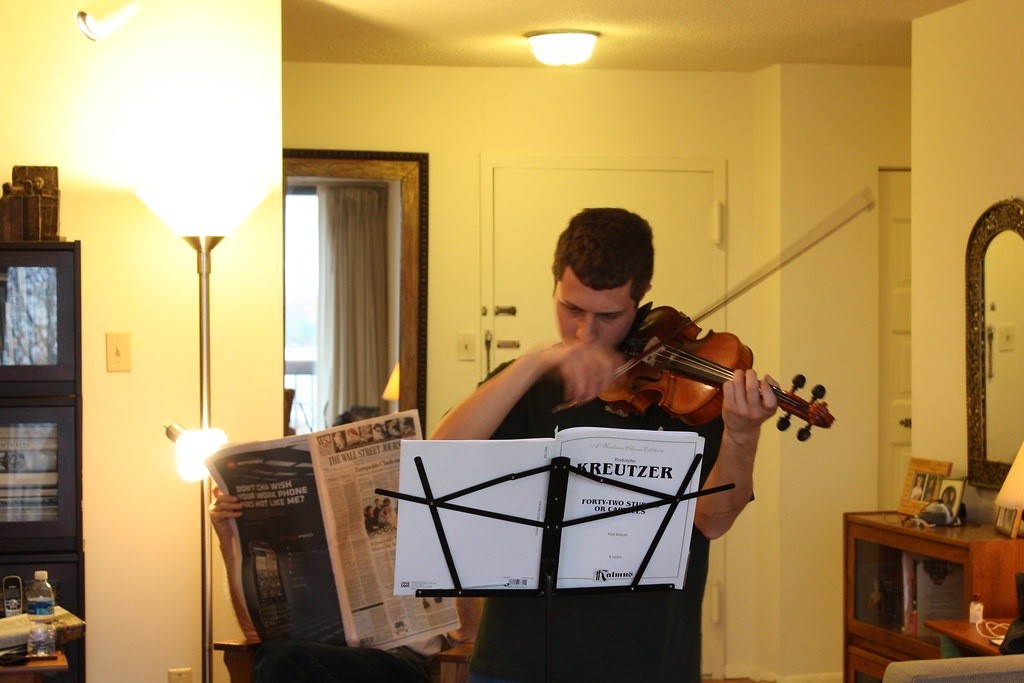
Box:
[936,474,968,522]
[897,457,953,517]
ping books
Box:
[392,425,706,597]
[0,422,58,522]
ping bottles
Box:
[968,593,983,624]
[26,570,56,657]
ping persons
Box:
[910,475,922,500]
[429,208,780,683]
[208,406,443,682]
[926,479,935,501]
[364,498,395,536]
[941,487,955,505]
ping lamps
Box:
[164,424,229,480]
[524,28,601,66]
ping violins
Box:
[603,301,836,441]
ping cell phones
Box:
[3,576,23,617]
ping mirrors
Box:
[966,201,1024,494]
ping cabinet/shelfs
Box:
[0,237,86,683]
[840,510,1023,682]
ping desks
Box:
[922,616,1015,658]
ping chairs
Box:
[212,593,483,683]
